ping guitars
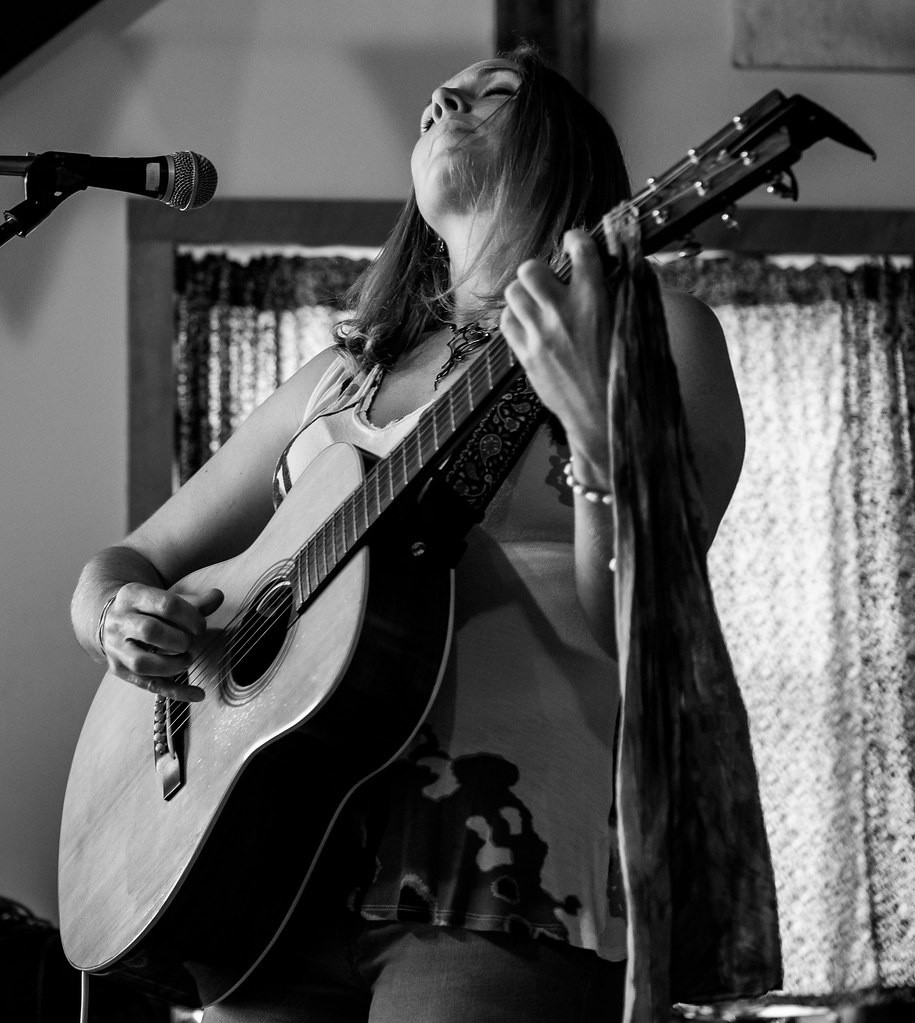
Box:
[58,88,879,1010]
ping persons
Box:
[67,45,747,1014]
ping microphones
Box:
[0,150,219,211]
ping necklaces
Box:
[433,320,502,392]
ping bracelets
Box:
[98,595,116,655]
[564,455,614,509]
